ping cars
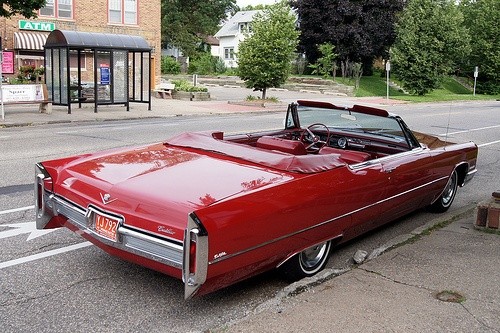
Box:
[31,98,480,305]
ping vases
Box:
[32,77,41,82]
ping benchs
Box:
[47,86,86,102]
[155,83,174,99]
[0,81,53,115]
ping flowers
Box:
[32,65,44,77]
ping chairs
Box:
[256,135,305,155]
[318,148,370,163]
